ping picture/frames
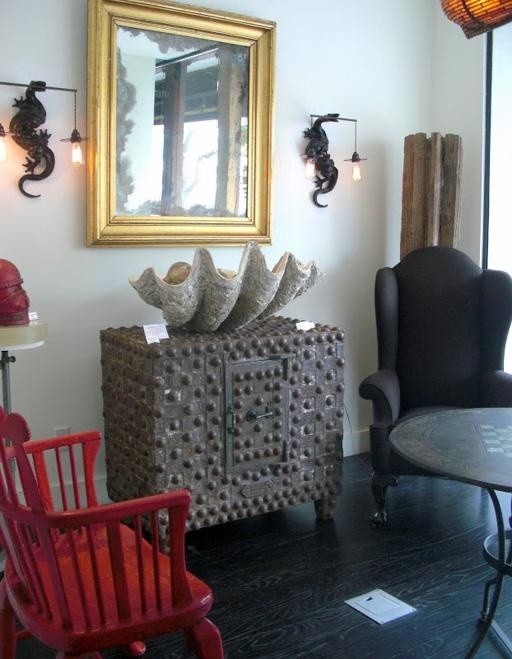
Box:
[85,0,279,248]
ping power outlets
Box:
[344,588,417,626]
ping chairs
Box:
[358,246,512,530]
[0,411,224,659]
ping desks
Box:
[0,324,45,576]
[389,407,512,659]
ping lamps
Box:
[441,0,512,39]
[300,115,316,180]
[0,80,29,164]
[338,117,367,183]
[45,86,90,168]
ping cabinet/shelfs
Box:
[98,315,343,557]
